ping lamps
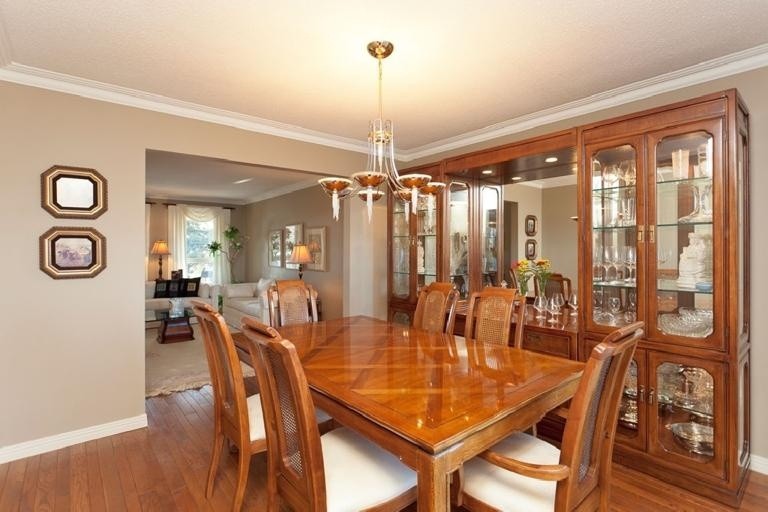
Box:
[319,40,445,229]
[150,241,172,281]
[288,245,313,279]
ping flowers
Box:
[512,260,532,296]
[530,260,552,292]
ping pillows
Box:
[153,277,201,298]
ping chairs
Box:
[412,282,460,334]
[268,280,318,328]
[239,317,418,511]
[451,318,646,512]
[190,300,334,511]
[464,286,536,438]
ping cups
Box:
[620,198,635,226]
[602,164,619,188]
[607,298,620,319]
[606,247,618,284]
[567,293,578,316]
[619,160,636,186]
[621,246,636,286]
[551,293,564,323]
[534,296,548,320]
[545,298,560,324]
[672,146,689,185]
[593,291,603,321]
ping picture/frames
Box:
[268,230,283,267]
[304,226,326,271]
[525,214,538,236]
[525,239,538,260]
[284,222,304,269]
[40,165,108,219]
[38,226,107,280]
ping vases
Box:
[536,279,547,315]
[518,284,528,318]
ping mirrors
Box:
[480,162,578,310]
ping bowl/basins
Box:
[659,314,710,335]
[665,422,713,455]
[679,306,717,324]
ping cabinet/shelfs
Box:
[386,172,478,331]
[579,88,752,507]
[447,303,577,447]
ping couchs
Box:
[222,281,315,328]
[145,280,219,321]
